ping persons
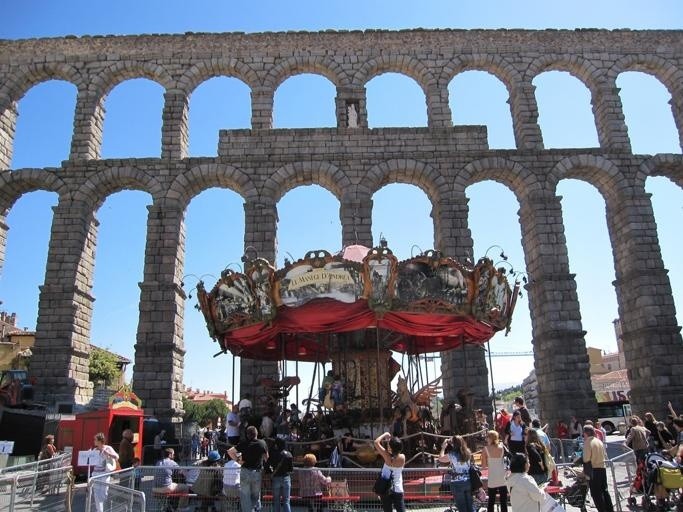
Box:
[89,432,119,512]
[154,396,683,511]
[37,434,56,491]
[119,429,139,469]
[131,458,142,491]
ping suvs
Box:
[596,401,633,434]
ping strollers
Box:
[326,480,351,511]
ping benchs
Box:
[151,492,361,511]
[378,487,565,512]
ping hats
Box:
[208,451,221,461]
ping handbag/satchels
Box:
[105,457,117,473]
[544,448,556,472]
[659,467,683,489]
[469,465,484,491]
[372,476,393,496]
